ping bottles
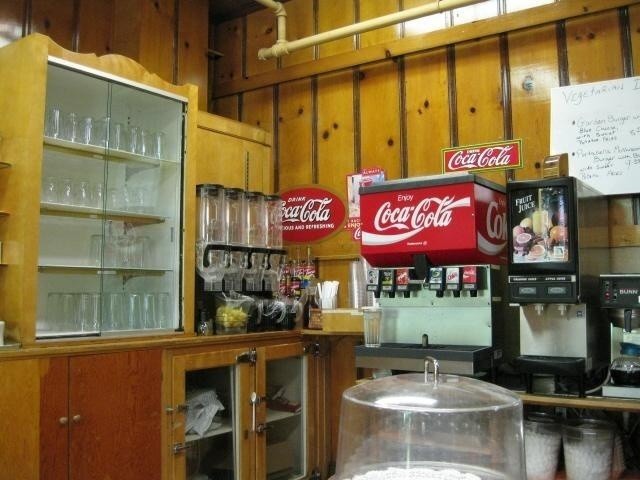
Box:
[302,287,318,328]
[282,243,316,299]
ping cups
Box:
[42,291,173,332]
[348,259,373,309]
[364,306,382,350]
[93,233,158,270]
[44,108,171,160]
[46,175,163,219]
[524,413,618,478]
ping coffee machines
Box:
[597,273,640,398]
